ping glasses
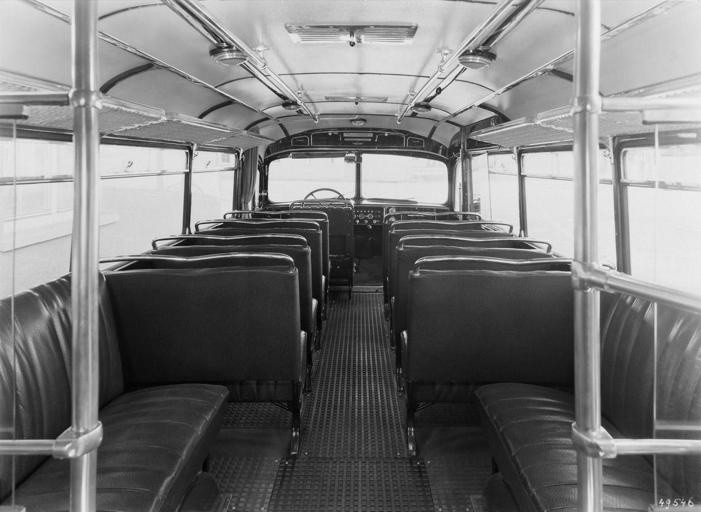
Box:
[459,49,495,71]
[409,100,434,115]
[210,48,248,67]
[347,115,369,127]
[283,98,303,114]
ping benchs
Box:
[0,267,229,511]
[96,193,357,456]
[471,269,701,512]
[376,198,580,457]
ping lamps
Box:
[283,98,303,114]
[347,115,369,127]
[409,100,434,115]
[210,48,248,67]
[459,49,495,71]
